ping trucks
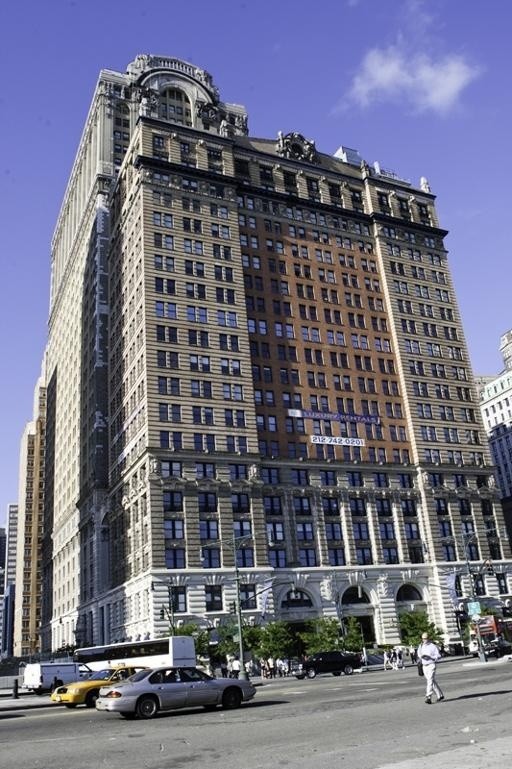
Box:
[22,661,99,697]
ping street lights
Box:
[199,529,280,692]
[419,525,509,664]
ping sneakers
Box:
[437,696,444,701]
[425,699,432,704]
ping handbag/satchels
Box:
[417,643,424,676]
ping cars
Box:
[92,665,259,718]
[483,640,512,660]
[49,661,150,709]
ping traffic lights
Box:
[227,598,235,616]
[159,609,165,621]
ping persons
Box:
[382,644,418,672]
[416,632,444,704]
[219,654,290,680]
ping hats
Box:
[421,632,429,639]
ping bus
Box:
[466,614,512,657]
[66,635,197,680]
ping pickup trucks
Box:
[291,650,361,679]
